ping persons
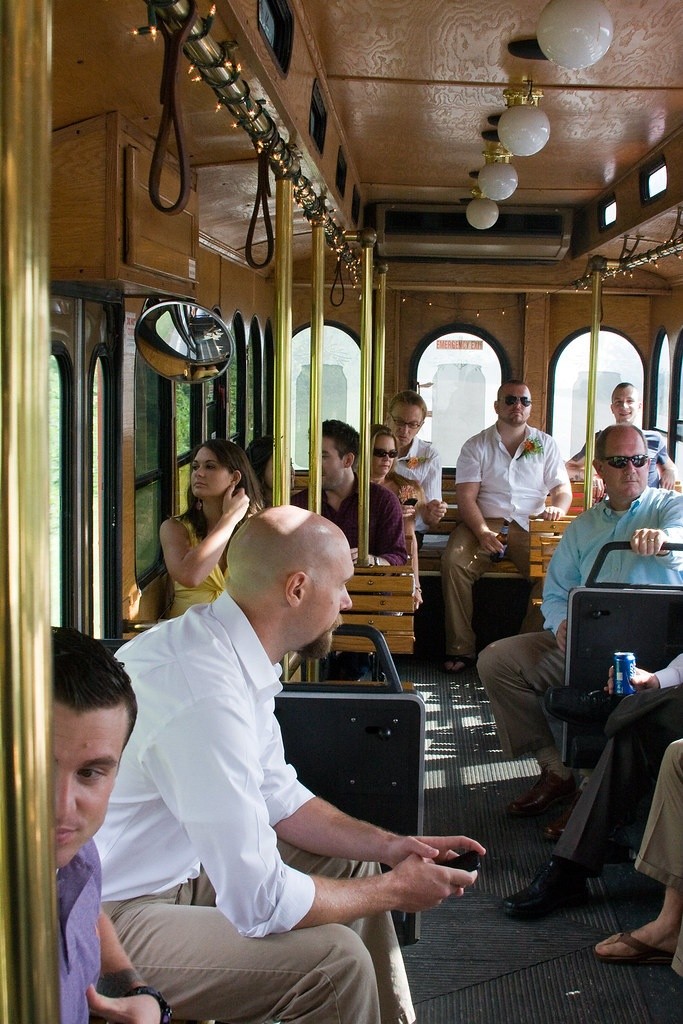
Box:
[563,382,677,506]
[441,378,572,672]
[159,438,267,623]
[92,502,486,1024]
[44,622,175,1024]
[591,736,683,977]
[383,390,447,552]
[501,645,683,923]
[247,434,297,510]
[477,421,683,853]
[356,423,426,612]
[289,419,409,576]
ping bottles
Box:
[489,520,510,564]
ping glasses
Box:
[389,412,422,429]
[596,454,649,469]
[374,448,398,458]
[499,395,532,407]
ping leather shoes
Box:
[546,685,630,734]
[506,767,577,818]
[543,798,578,839]
[505,857,594,919]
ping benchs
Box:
[277,470,618,682]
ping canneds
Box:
[611,651,640,696]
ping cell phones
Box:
[404,498,418,506]
[438,850,482,872]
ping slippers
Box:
[444,652,476,673]
[594,929,675,964]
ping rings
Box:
[647,538,654,542]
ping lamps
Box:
[462,3,615,231]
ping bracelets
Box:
[374,555,381,566]
[123,985,173,1024]
[414,586,423,593]
